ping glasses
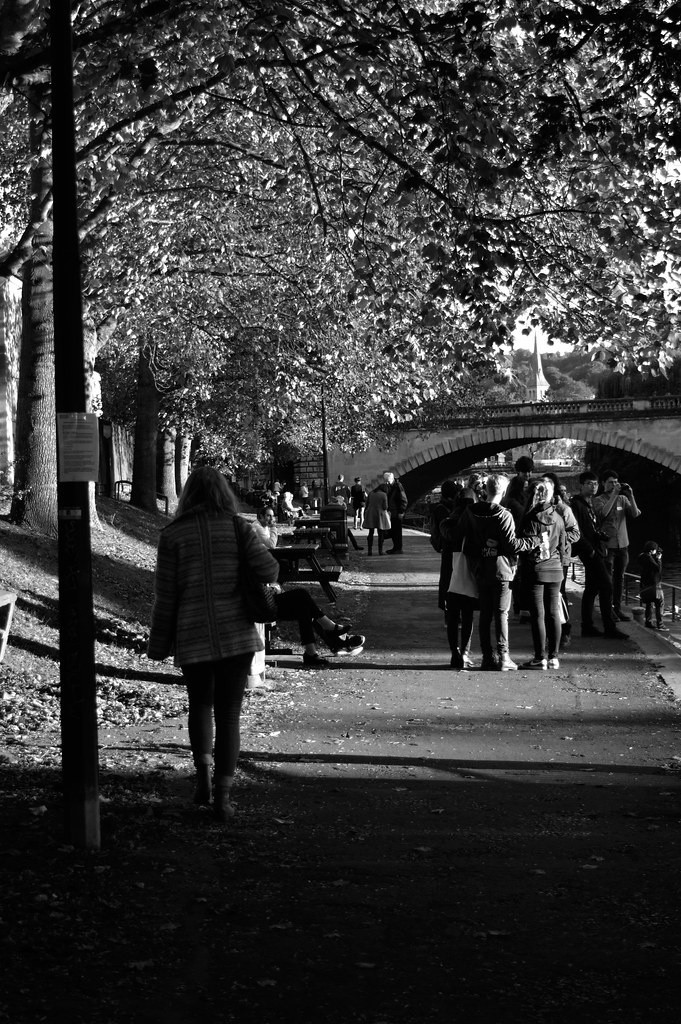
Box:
[584,482,599,487]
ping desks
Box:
[267,515,345,602]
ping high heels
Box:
[451,653,461,667]
[461,653,475,668]
[520,610,532,624]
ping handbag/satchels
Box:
[558,591,570,624]
[233,515,277,623]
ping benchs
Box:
[334,542,348,554]
[323,564,343,582]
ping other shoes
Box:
[645,621,657,628]
[334,633,365,651]
[386,547,403,555]
[614,607,633,621]
[326,623,352,638]
[581,626,604,637]
[333,646,363,657]
[355,546,363,550]
[560,635,571,647]
[658,624,669,631]
[603,626,630,640]
[379,550,385,555]
[303,652,329,666]
[368,547,372,555]
[611,608,621,622]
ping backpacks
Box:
[430,503,451,553]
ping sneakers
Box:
[520,658,547,670]
[548,658,559,669]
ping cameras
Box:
[596,531,611,542]
[620,484,629,490]
[657,547,663,555]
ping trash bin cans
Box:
[318,505,347,548]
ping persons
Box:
[148,464,280,824]
[428,454,670,670]
[247,472,406,664]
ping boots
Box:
[497,643,518,671]
[480,643,499,669]
